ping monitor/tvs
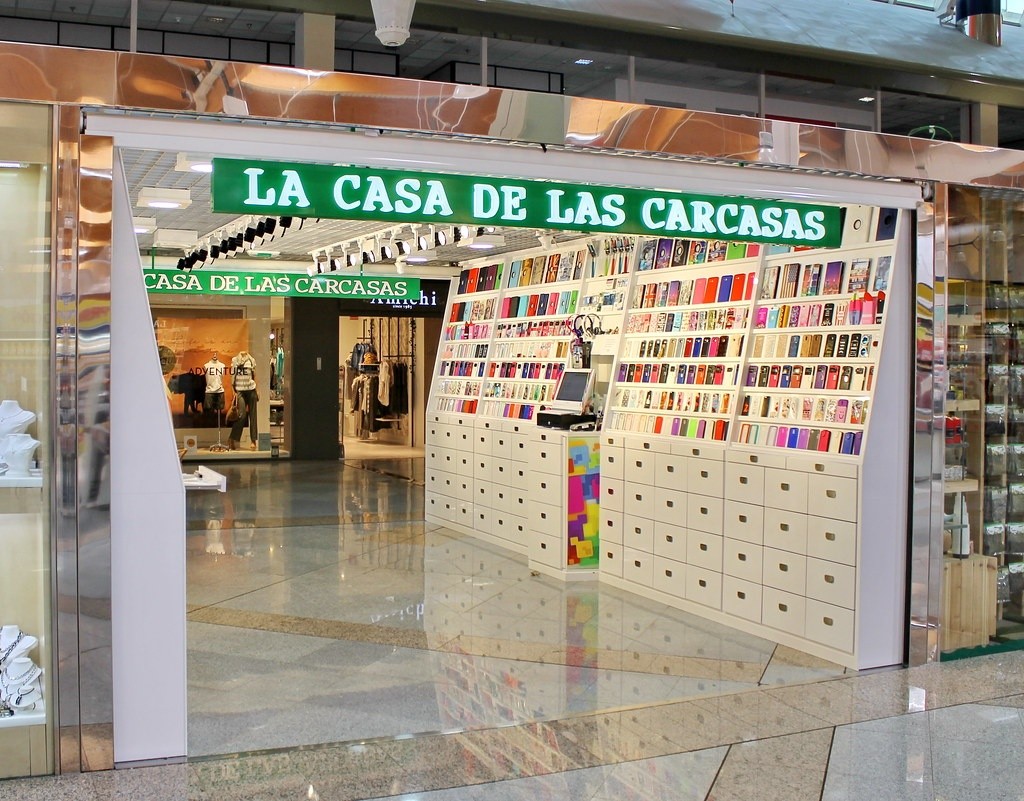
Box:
[551,368,596,412]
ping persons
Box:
[202,357,225,409]
[227,351,258,450]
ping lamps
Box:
[934,0,1001,46]
[177,213,500,277]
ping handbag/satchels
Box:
[226,393,239,422]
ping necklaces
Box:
[0,627,24,666]
[12,439,34,454]
[1,664,38,688]
[0,409,23,422]
[15,688,34,705]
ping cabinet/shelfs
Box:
[2,98,57,780]
[602,209,910,671]
[423,237,637,582]
[935,277,998,653]
